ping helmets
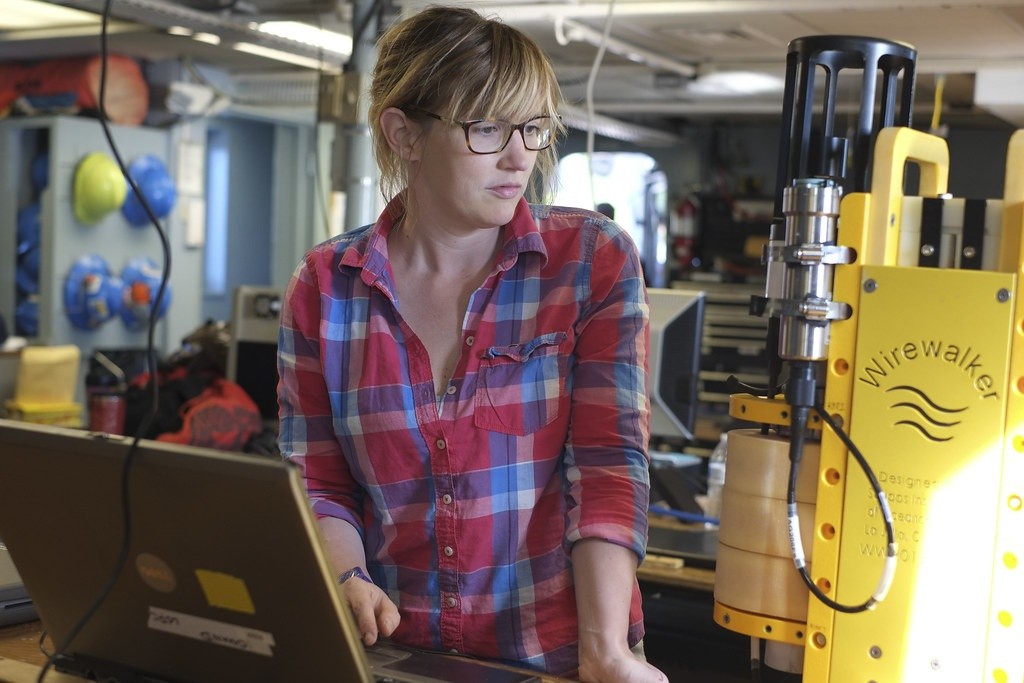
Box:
[73,151,127,228]
[120,259,171,333]
[123,155,176,227]
[65,255,124,329]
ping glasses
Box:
[399,105,563,154]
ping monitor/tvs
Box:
[641,287,705,469]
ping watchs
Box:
[337,566,372,586]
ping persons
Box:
[596,203,648,288]
[277,7,671,683]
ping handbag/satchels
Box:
[124,367,264,453]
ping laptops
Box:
[1,417,543,683]
